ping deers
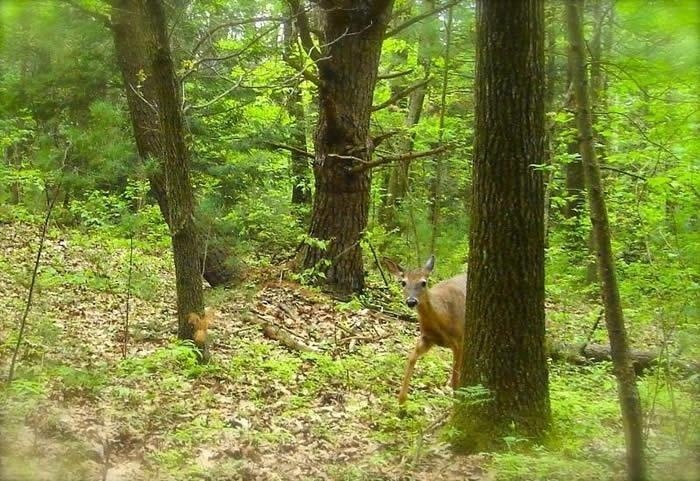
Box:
[383,254,468,404]
